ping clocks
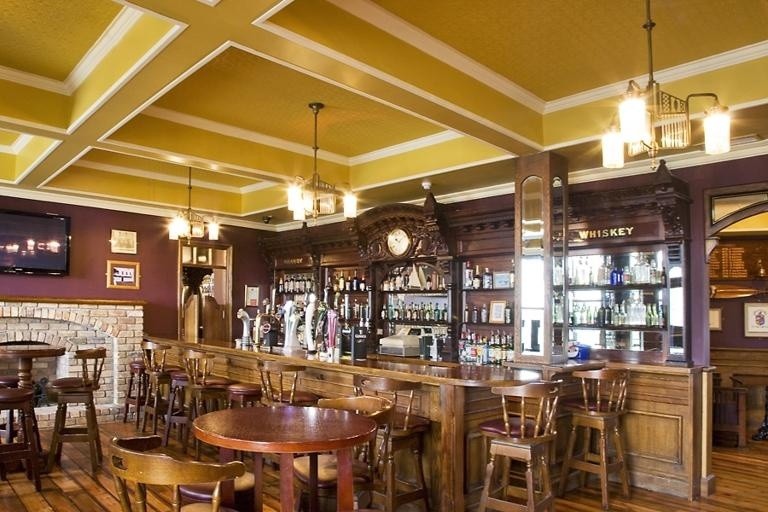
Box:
[385,225,414,259]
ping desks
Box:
[191,406,378,511]
[0,343,66,474]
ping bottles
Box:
[593,328,660,352]
[552,253,669,327]
[272,255,515,368]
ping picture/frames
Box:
[106,259,140,290]
[111,229,137,255]
[744,302,768,337]
[709,308,722,330]
[244,284,260,308]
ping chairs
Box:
[173,385,257,512]
[479,378,563,512]
[108,432,247,510]
[560,367,631,511]
[292,393,395,510]
[45,346,106,472]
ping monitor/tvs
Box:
[0,209,70,276]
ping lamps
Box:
[286,101,358,222]
[601,0,731,171]
[167,167,219,246]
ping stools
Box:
[140,341,183,435]
[352,372,433,511]
[162,368,210,448]
[227,382,262,464]
[0,388,41,491]
[183,346,233,462]
[123,360,159,427]
[256,358,318,472]
